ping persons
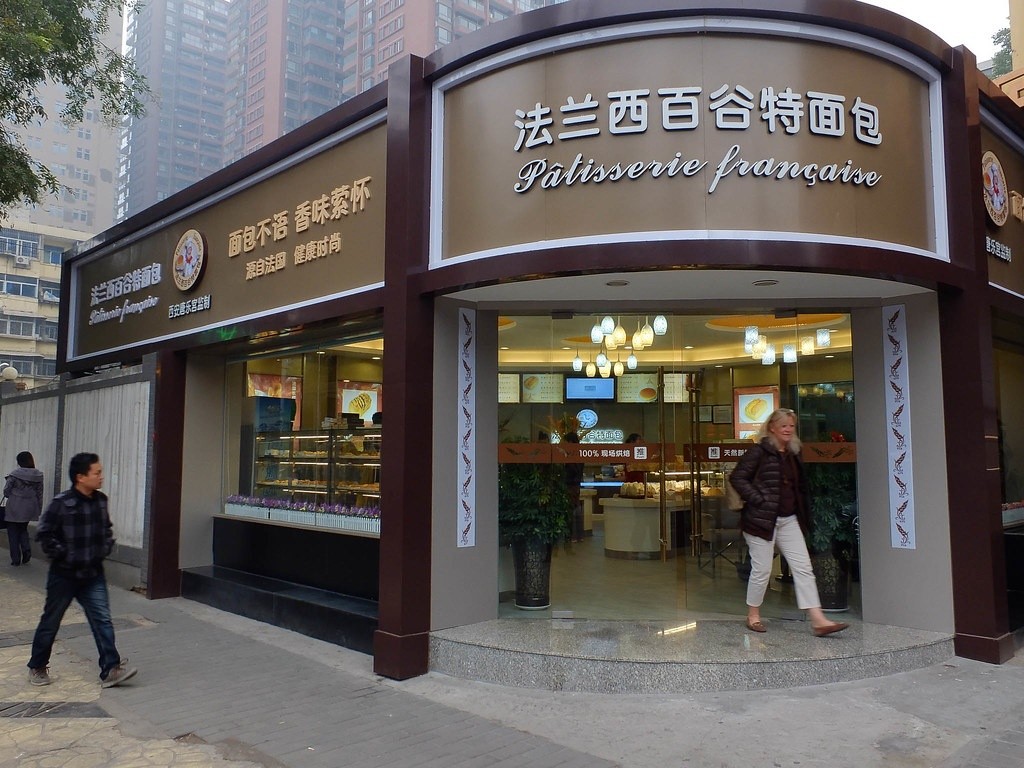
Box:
[3,451,44,566]
[729,408,849,636]
[559,432,585,543]
[27,452,138,688]
[623,433,647,483]
[539,430,549,443]
[363,412,383,482]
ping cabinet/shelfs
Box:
[253,430,381,507]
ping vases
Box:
[270,508,316,526]
[316,513,381,534]
[547,430,561,444]
[224,503,269,519]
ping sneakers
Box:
[28,668,51,685]
[102,667,137,688]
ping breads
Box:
[639,388,657,400]
[745,399,767,421]
[620,481,723,498]
[524,376,538,390]
[349,393,371,415]
[257,449,380,492]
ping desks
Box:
[601,498,720,560]
[577,489,597,537]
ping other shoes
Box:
[814,622,849,637]
[746,617,766,633]
[22,549,31,564]
[11,561,20,566]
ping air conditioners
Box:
[15,255,30,266]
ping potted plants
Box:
[497,436,572,607]
[799,470,849,610]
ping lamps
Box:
[783,338,797,363]
[744,316,776,365]
[572,348,582,371]
[586,316,667,379]
[817,328,831,346]
[801,337,815,356]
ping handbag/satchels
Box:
[0,495,7,530]
[724,472,744,512]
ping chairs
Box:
[694,496,741,578]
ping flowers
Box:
[226,495,381,518]
[539,414,564,435]
[692,405,713,422]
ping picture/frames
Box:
[713,405,732,424]
[335,383,383,428]
